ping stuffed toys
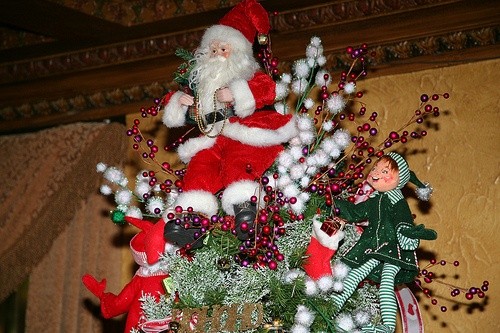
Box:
[324,152,438,333]
[160,0,295,251]
[82,210,189,333]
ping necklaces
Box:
[195,86,229,138]
